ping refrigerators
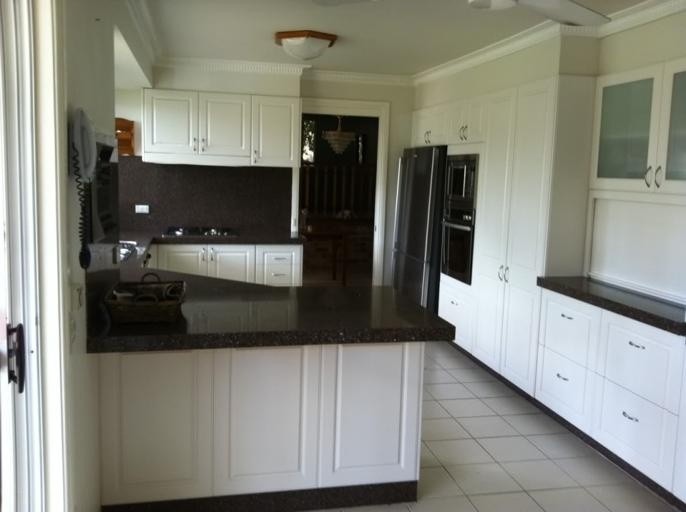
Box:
[389,146,445,319]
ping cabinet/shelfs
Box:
[250,94,301,168]
[533,289,686,511]
[589,58,686,206]
[470,78,596,400]
[410,95,483,148]
[435,272,470,352]
[157,244,303,288]
[140,86,252,168]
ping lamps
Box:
[275,31,338,62]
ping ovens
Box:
[438,153,480,286]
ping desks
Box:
[303,209,356,286]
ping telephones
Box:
[70,107,98,184]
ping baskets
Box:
[102,272,187,322]
